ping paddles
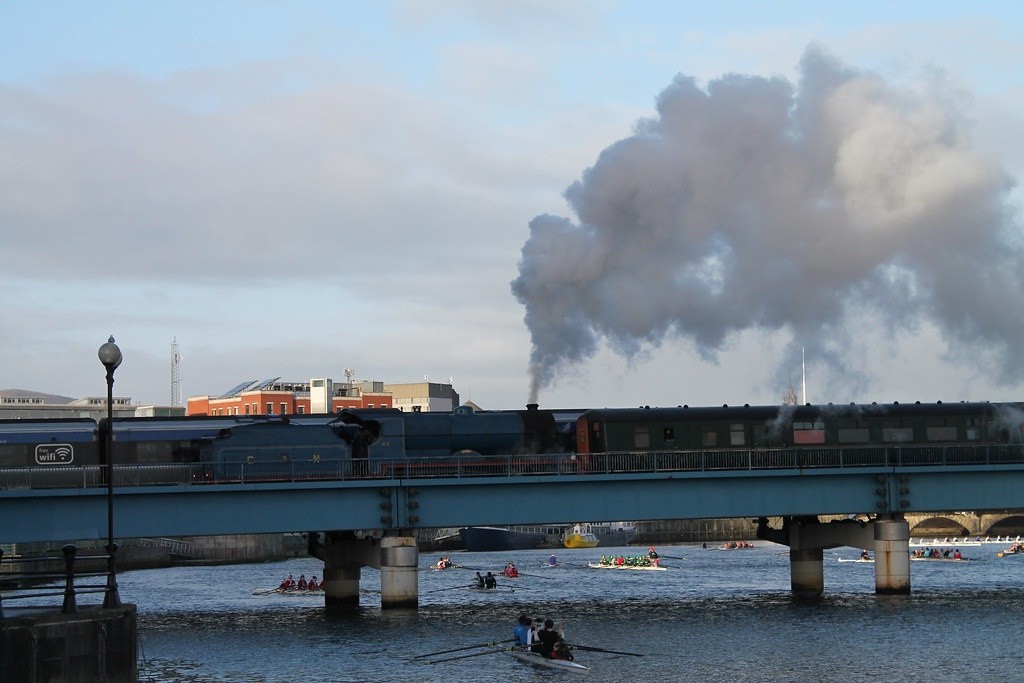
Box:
[473,573,504,580]
[496,584,524,588]
[659,555,686,560]
[412,639,517,659]
[426,582,477,593]
[566,643,608,651]
[517,573,551,579]
[568,646,646,657]
[425,642,544,665]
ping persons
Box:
[724,541,753,549]
[280,574,324,591]
[513,616,565,659]
[547,545,660,567]
[861,550,869,560]
[437,557,518,588]
[913,542,1024,558]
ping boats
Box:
[469,587,515,593]
[586,561,667,571]
[909,557,971,563]
[275,587,326,595]
[838,558,876,562]
[500,647,590,676]
[428,564,464,569]
[1002,550,1015,554]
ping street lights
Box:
[97,335,123,607]
